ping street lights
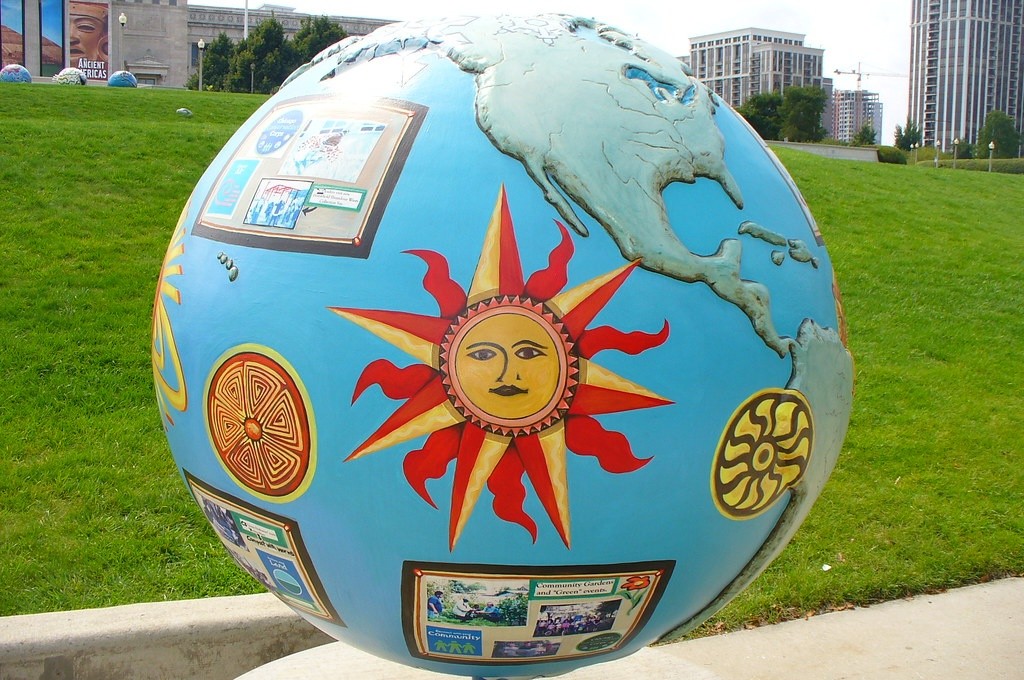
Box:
[910,144,914,156]
[250,63,255,94]
[988,141,994,171]
[954,138,959,169]
[915,142,919,163]
[119,13,126,70]
[936,140,941,168]
[198,39,205,91]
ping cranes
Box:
[833,61,904,133]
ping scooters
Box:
[544,617,579,636]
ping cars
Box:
[517,641,547,656]
[504,644,519,654]
